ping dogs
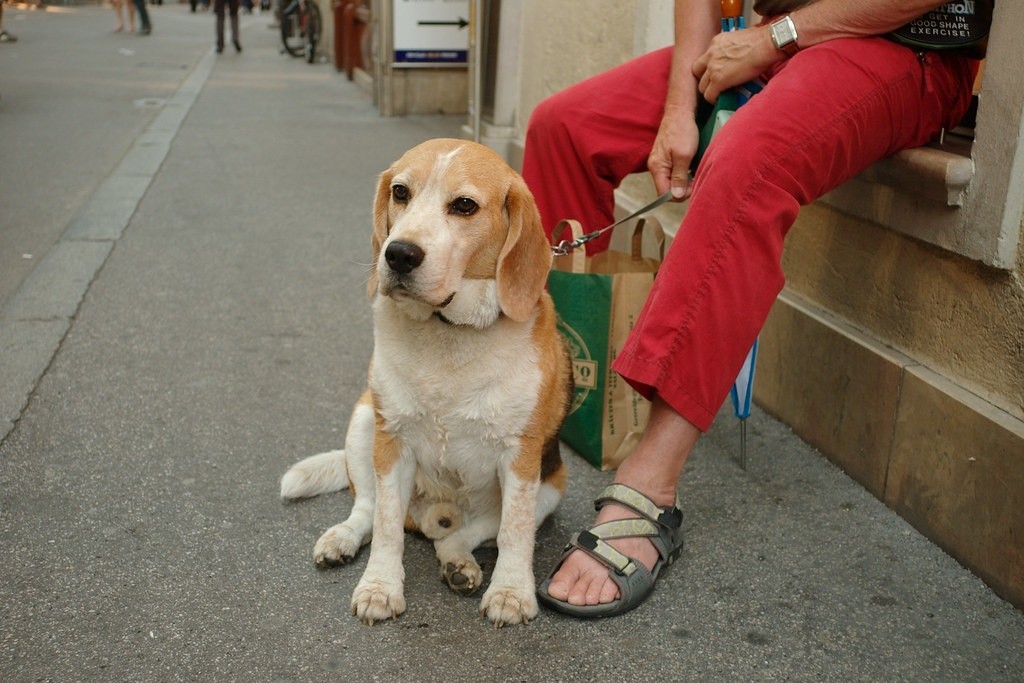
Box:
[280,136,576,630]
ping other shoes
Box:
[112,25,123,32]
[217,46,223,53]
[0,31,17,42]
[233,39,241,53]
[127,23,135,33]
[141,23,151,35]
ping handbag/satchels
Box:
[892,0,995,49]
[547,216,667,472]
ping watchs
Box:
[769,14,801,58]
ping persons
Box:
[112,0,151,35]
[523,0,995,618]
[213,0,242,54]
[0,0,18,42]
[150,0,272,15]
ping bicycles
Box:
[281,0,322,63]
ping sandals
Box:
[538,484,685,618]
